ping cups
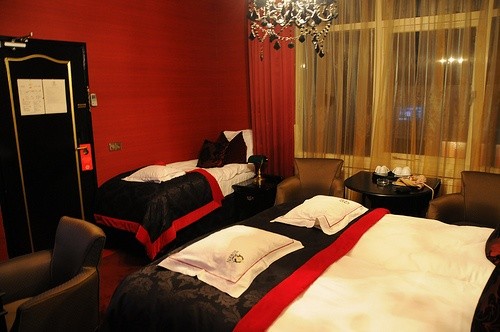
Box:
[381,166,390,174]
[392,167,402,175]
[375,166,382,173]
[401,167,412,175]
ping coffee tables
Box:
[343,171,441,218]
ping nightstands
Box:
[232,174,283,220]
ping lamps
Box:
[248,154,269,182]
[247,0,339,62]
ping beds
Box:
[105,208,500,332]
[94,129,259,264]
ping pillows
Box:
[196,139,230,167]
[156,225,305,299]
[270,194,369,236]
[121,165,186,184]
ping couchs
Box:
[275,157,344,206]
[0,217,106,332]
[426,170,500,239]
